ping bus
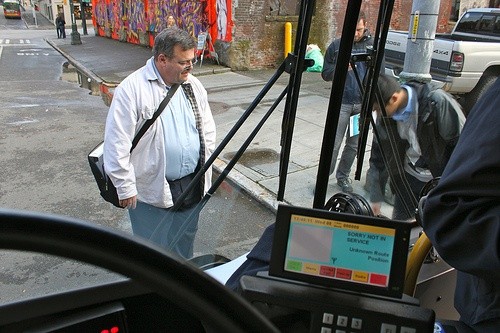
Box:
[3,0,21,19]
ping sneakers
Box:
[338,180,354,193]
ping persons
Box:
[103,27,217,260]
[313,11,385,194]
[364,74,467,221]
[422,76,500,333]
[56,14,66,38]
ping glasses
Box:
[167,53,199,70]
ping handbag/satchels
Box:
[162,172,202,212]
[88,141,124,209]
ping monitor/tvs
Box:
[269,204,410,300]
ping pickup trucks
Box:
[378,8,500,118]
